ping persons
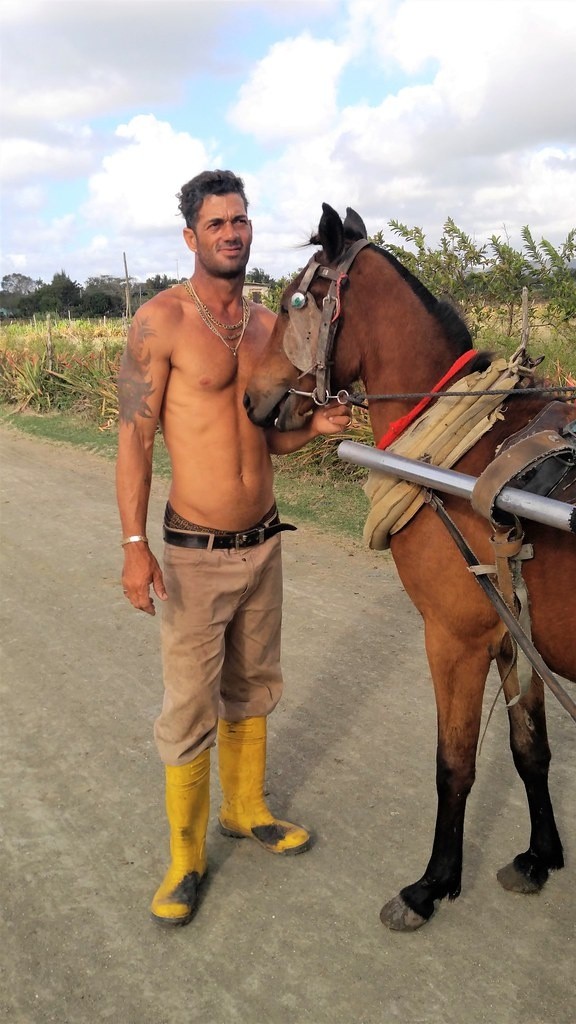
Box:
[115,170,353,926]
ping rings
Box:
[123,590,127,594]
[345,418,352,426]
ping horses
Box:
[241,201,576,933]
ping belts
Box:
[163,511,298,550]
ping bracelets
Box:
[122,535,149,549]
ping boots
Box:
[151,747,211,925]
[217,715,312,855]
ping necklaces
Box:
[183,280,251,357]
